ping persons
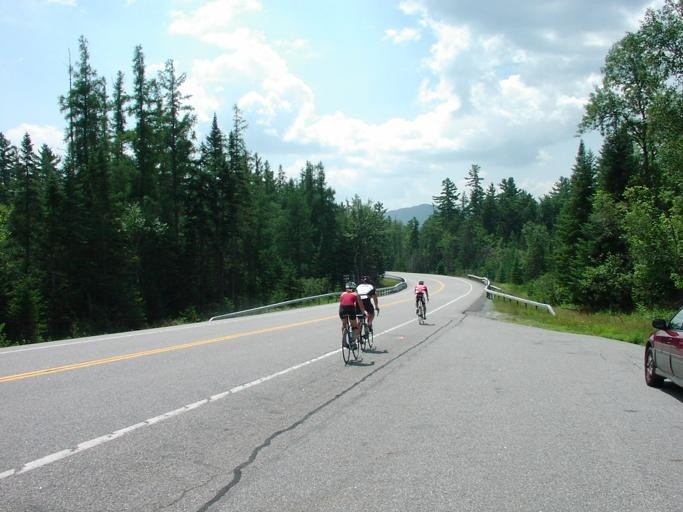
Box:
[355,276,379,342]
[414,281,429,319]
[338,281,367,349]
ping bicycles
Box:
[417,296,429,325]
[360,308,380,350]
[341,313,366,364]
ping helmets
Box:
[360,275,369,283]
[345,281,357,289]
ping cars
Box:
[644,305,683,392]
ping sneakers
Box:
[416,308,426,319]
